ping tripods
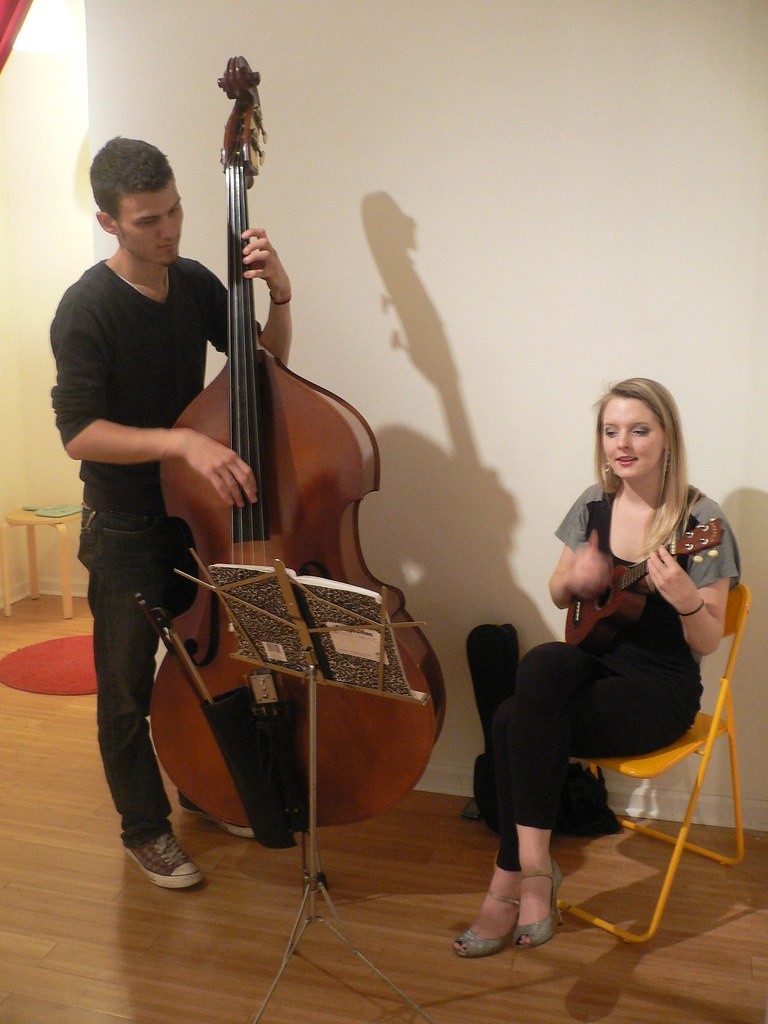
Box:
[173,547,435,1024]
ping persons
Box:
[49,135,293,887]
[452,378,743,957]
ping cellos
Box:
[148,56,447,831]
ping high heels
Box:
[514,859,563,947]
[454,889,520,956]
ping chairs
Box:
[486,578,751,944]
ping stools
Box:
[0,509,84,621]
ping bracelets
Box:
[680,599,704,617]
[269,291,293,305]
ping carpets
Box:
[0,634,98,695]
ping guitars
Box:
[565,518,725,651]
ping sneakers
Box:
[178,788,255,837]
[126,832,202,888]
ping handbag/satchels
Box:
[474,753,621,837]
